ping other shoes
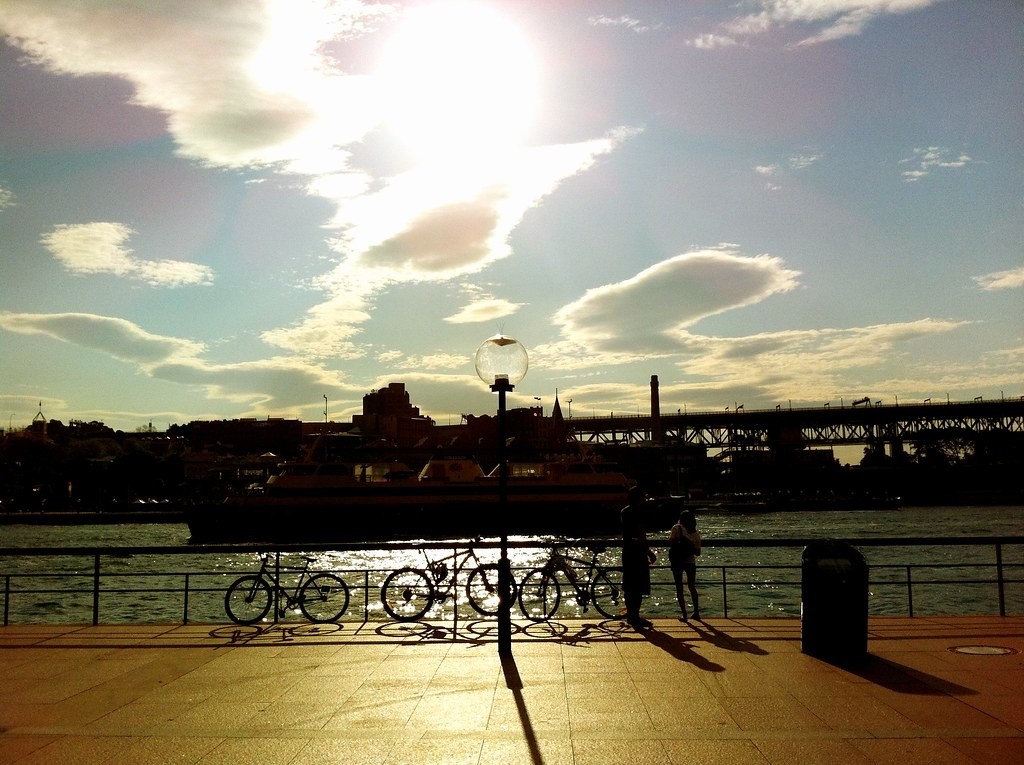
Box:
[627,617,632,624]
[691,615,701,620]
[679,617,688,622]
[632,619,652,627]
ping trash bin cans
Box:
[799,538,869,657]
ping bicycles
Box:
[381,535,518,622]
[519,538,631,623]
[225,547,350,626]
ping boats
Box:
[177,434,661,545]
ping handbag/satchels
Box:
[671,526,696,562]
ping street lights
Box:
[474,334,530,652]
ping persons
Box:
[669,509,701,622]
[620,485,657,626]
